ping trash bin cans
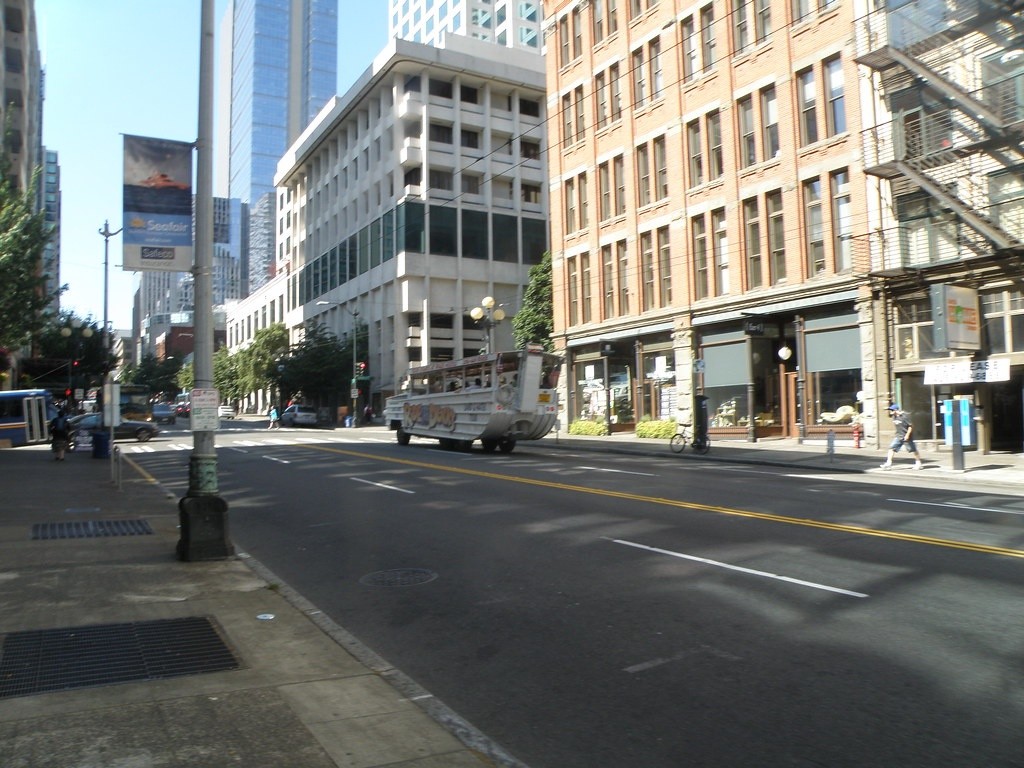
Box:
[91,431,110,459]
[342,416,352,428]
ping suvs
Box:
[96,384,153,423]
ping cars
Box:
[154,405,176,424]
[218,405,235,420]
[280,405,317,426]
[171,402,191,417]
[66,412,160,442]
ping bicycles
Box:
[52,427,67,462]
[671,422,710,454]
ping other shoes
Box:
[60,458,64,461]
[912,464,924,470]
[268,427,271,430]
[275,427,279,430]
[56,456,59,461]
[880,463,891,471]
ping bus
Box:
[384,342,562,453]
[0,390,59,449]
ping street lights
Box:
[316,302,358,427]
[62,318,93,412]
[470,297,504,354]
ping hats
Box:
[888,404,900,411]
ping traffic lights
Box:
[73,358,80,373]
[65,388,71,397]
[356,362,364,375]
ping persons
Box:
[448,374,518,391]
[46,410,71,461]
[93,406,97,412]
[267,405,280,430]
[879,404,922,470]
[362,402,375,424]
